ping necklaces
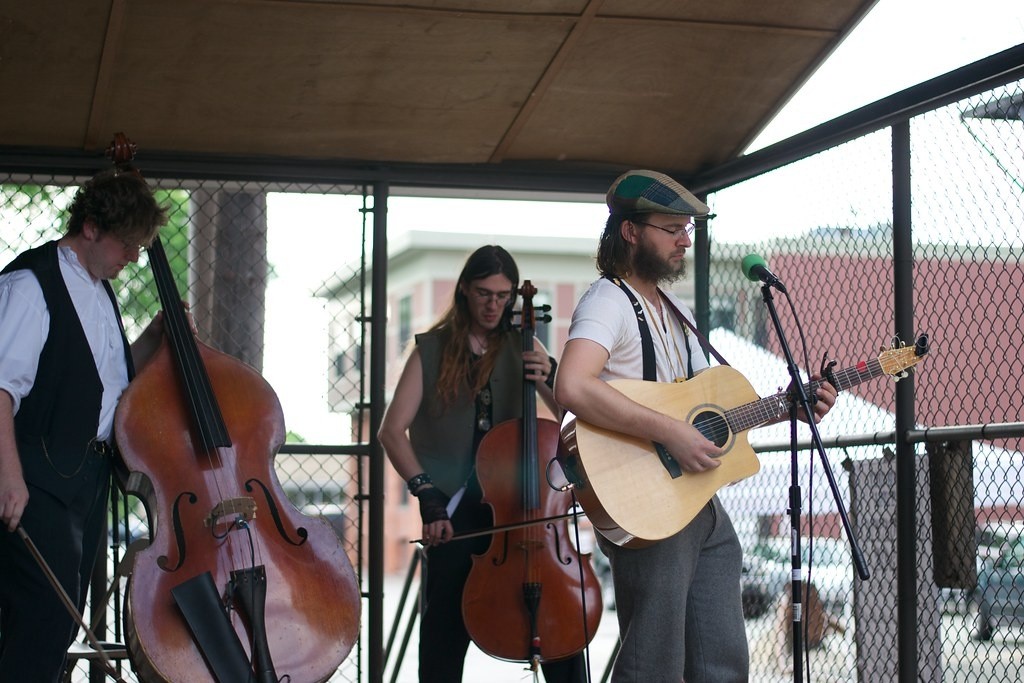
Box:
[469,327,492,356]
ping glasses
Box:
[472,296,514,306]
[636,221,695,239]
[114,232,149,252]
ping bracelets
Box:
[406,473,435,493]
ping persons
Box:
[375,246,588,683]
[553,169,837,683]
[0,167,199,683]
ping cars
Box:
[592,535,774,621]
[744,538,860,610]
[965,535,1023,641]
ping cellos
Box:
[460,280,601,683]
[105,130,359,683]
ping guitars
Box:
[555,334,931,547]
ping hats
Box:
[607,170,710,217]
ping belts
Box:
[93,441,112,455]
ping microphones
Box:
[742,254,787,293]
